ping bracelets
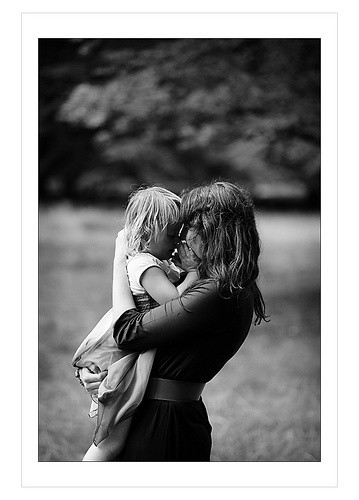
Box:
[75,367,85,388]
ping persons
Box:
[72,185,204,462]
[74,178,285,461]
